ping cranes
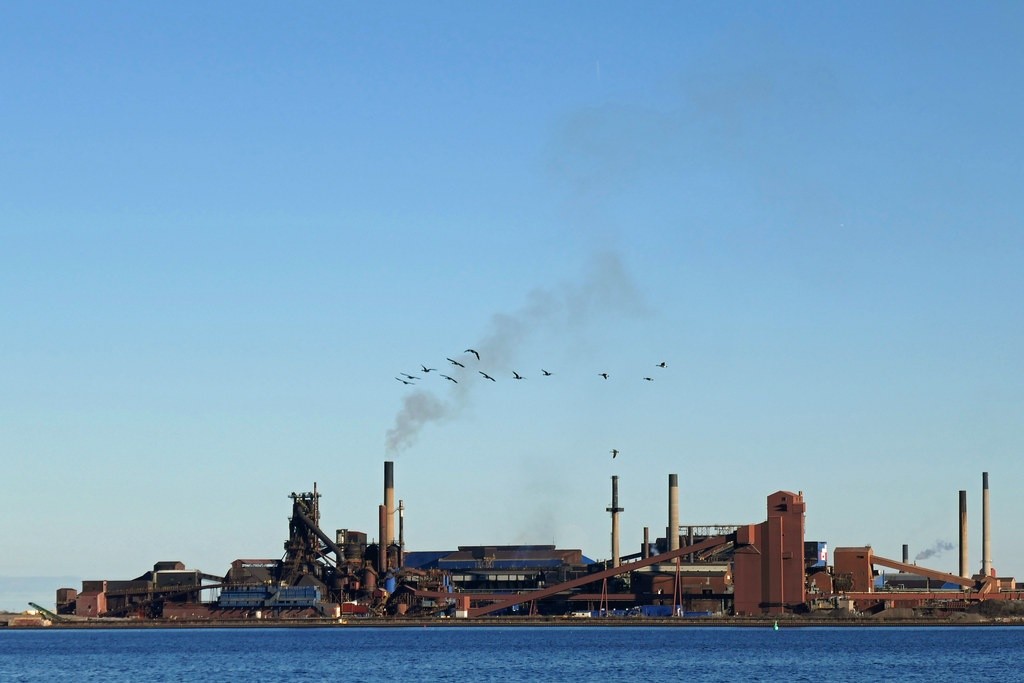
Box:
[29,602,67,624]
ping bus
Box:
[8,617,52,627]
[571,611,592,618]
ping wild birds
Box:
[394,347,668,386]
[608,447,620,459]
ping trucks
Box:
[627,605,682,617]
